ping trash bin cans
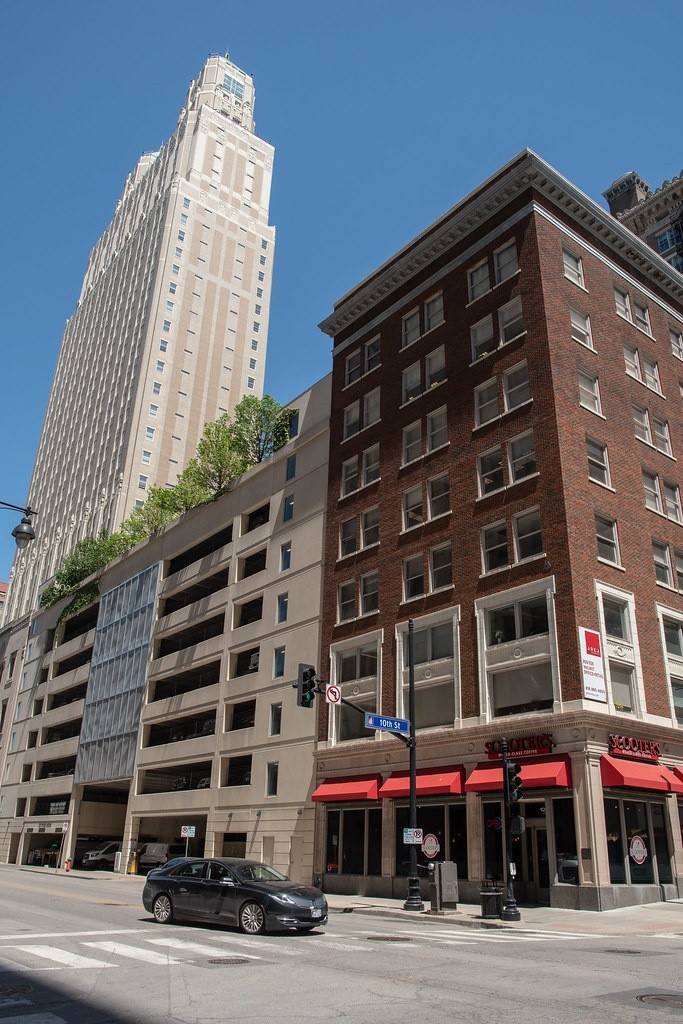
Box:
[479,886,504,918]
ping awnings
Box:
[311,773,383,802]
[379,764,466,798]
[464,753,572,792]
[600,753,683,795]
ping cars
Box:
[142,857,328,935]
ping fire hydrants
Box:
[65,857,72,872]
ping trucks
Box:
[137,843,191,875]
[82,840,143,871]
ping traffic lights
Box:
[507,763,523,803]
[297,663,316,708]
